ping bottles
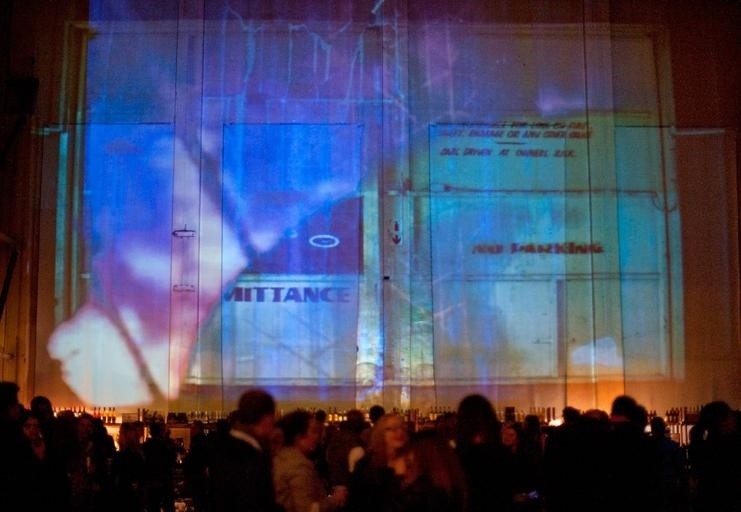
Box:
[170,437,188,483]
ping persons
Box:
[2,381,739,511]
